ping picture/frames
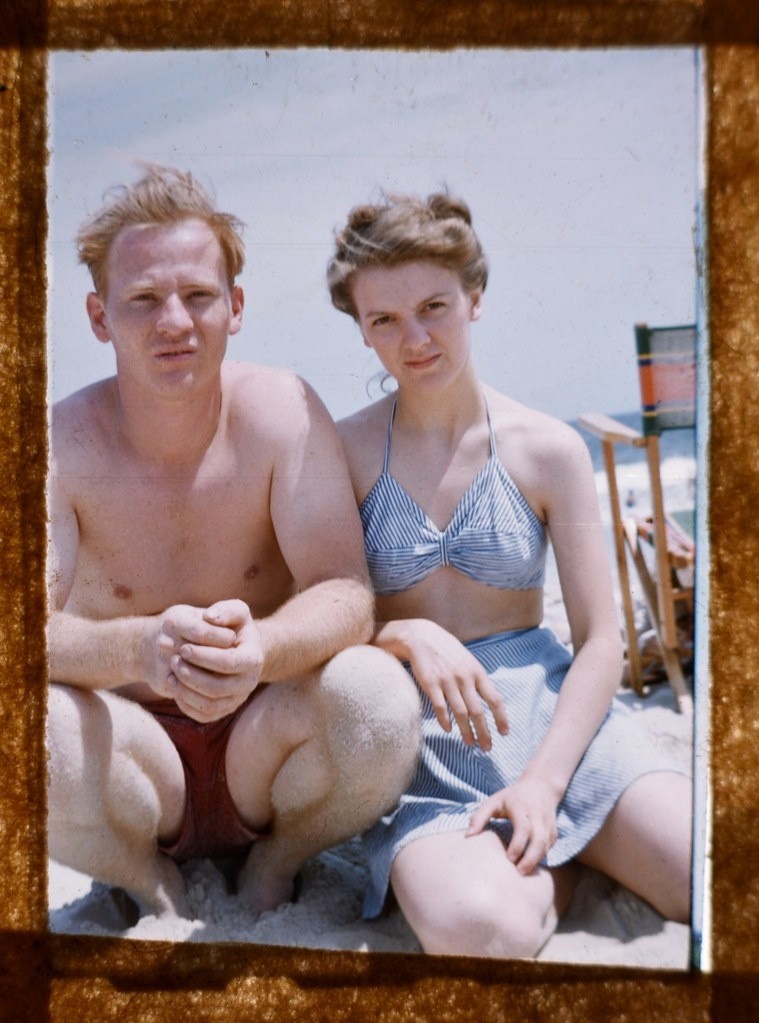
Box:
[0,0,754,1022]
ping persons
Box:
[39,157,424,940]
[319,185,702,961]
